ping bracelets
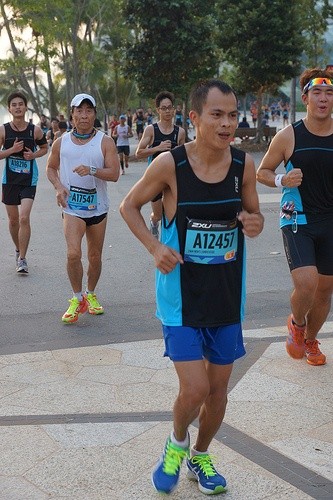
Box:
[89,165,97,176]
[275,174,285,188]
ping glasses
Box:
[159,106,174,110]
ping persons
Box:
[261,119,271,142]
[174,104,184,128]
[116,78,265,495]
[0,93,48,274]
[257,67,333,365]
[35,113,74,147]
[250,100,291,128]
[45,94,120,323]
[132,107,148,141]
[124,109,133,129]
[106,113,133,175]
[186,112,190,129]
[238,117,251,140]
[146,107,155,125]
[134,90,187,240]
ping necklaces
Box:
[72,127,96,145]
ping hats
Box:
[119,115,126,120]
[70,93,97,113]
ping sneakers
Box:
[17,257,28,273]
[286,313,307,359]
[304,330,327,365]
[152,428,190,494]
[187,446,227,494]
[16,250,20,265]
[61,295,89,323]
[84,291,105,314]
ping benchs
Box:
[234,127,276,143]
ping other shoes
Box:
[150,213,159,240]
